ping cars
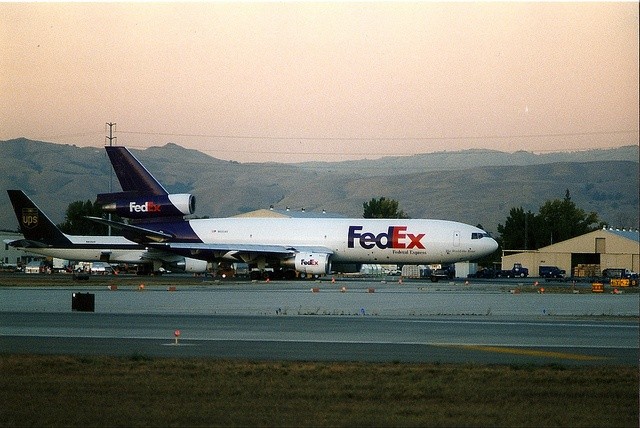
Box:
[90,261,106,273]
[24,260,47,273]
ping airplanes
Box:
[3,189,209,274]
[79,146,499,283]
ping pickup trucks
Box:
[72,261,89,272]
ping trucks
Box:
[497,263,529,278]
[602,268,638,278]
[538,266,566,281]
